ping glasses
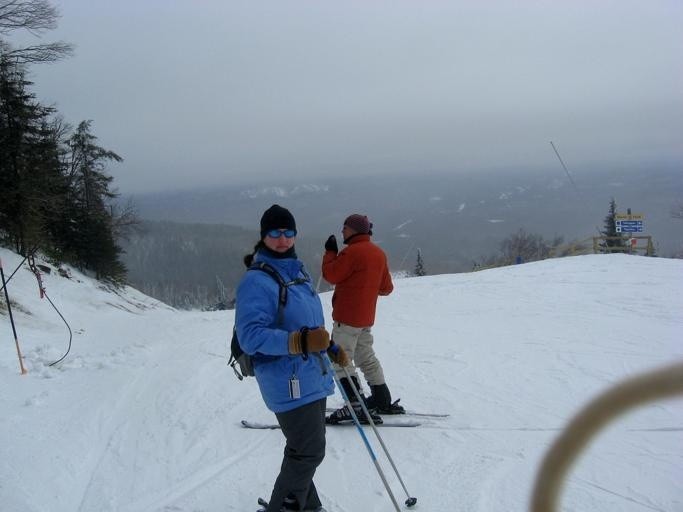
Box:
[268,229,297,239]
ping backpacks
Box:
[231,262,288,377]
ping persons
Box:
[235,205,348,511]
[320,213,394,420]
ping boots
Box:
[365,384,391,409]
[331,393,365,419]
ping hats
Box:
[344,214,370,234]
[261,204,296,238]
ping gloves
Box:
[328,340,348,366]
[325,234,338,253]
[290,326,329,355]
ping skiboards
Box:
[241,406,451,430]
